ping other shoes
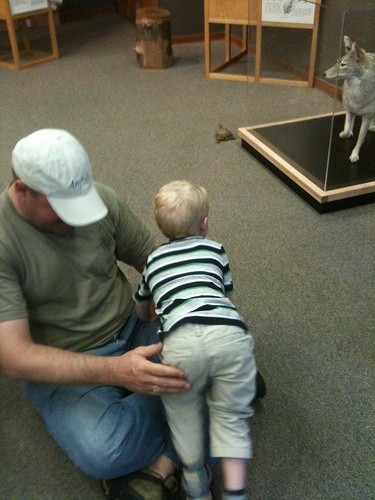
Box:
[103,466,178,500]
[220,489,246,500]
[181,461,211,500]
[255,369,266,398]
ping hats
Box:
[11,128,108,227]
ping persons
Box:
[0,127,269,480]
[131,179,257,499]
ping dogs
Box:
[322,35,375,162]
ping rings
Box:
[152,385,160,394]
